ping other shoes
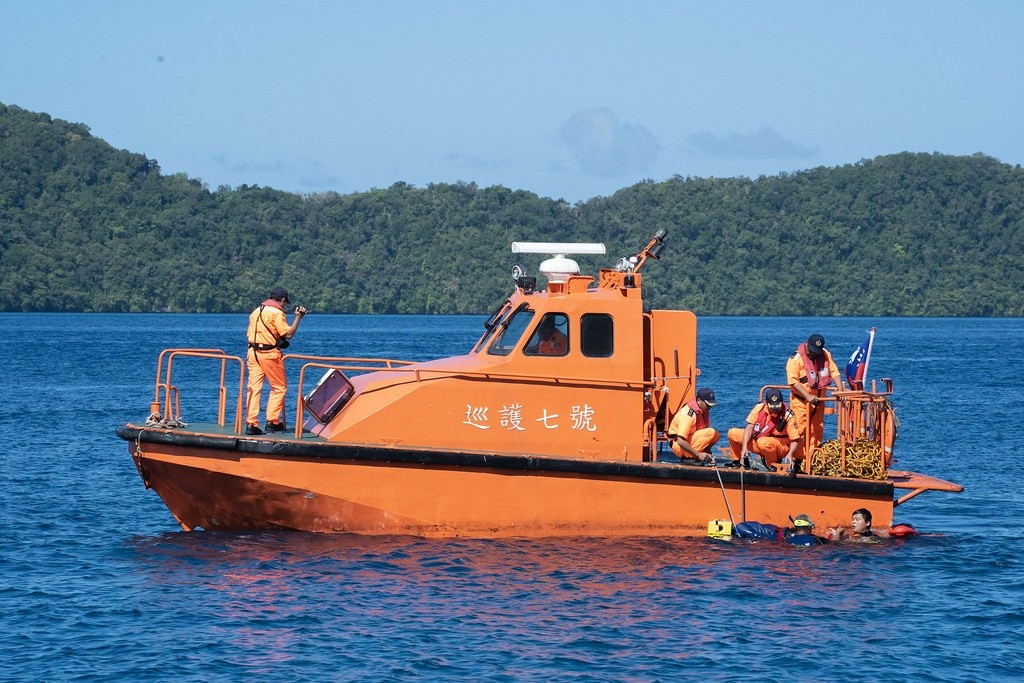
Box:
[725,459,751,469]
[265,421,284,431]
[680,456,704,467]
[246,423,262,434]
[760,456,777,471]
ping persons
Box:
[786,333,847,474]
[537,313,568,355]
[787,514,845,547]
[724,387,800,472]
[829,508,885,543]
[245,287,308,436]
[667,388,721,466]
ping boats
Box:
[115,227,967,544]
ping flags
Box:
[844,330,876,391]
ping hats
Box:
[808,334,825,354]
[767,388,784,409]
[697,388,717,408]
[269,286,291,304]
